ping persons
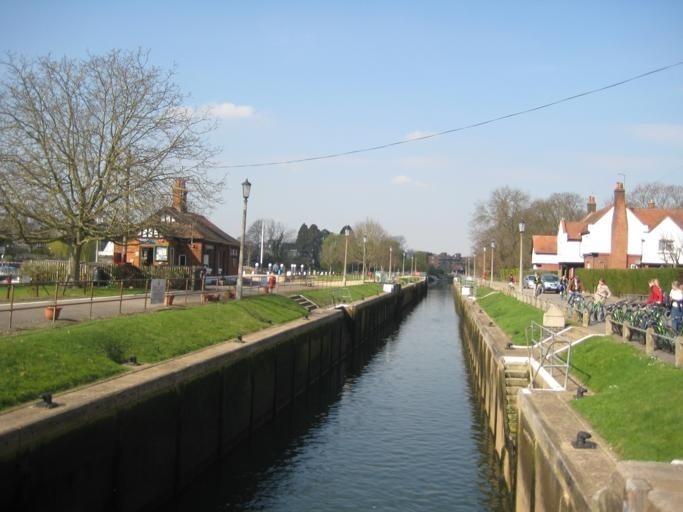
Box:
[642,278,683,332]
[267,273,276,294]
[558,274,611,309]
[534,271,543,297]
[508,274,514,293]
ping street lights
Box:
[341,224,349,287]
[234,178,251,299]
[517,219,525,293]
[361,235,366,282]
[489,242,495,289]
[463,246,486,286]
[387,245,417,278]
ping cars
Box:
[523,273,535,289]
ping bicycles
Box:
[568,292,682,353]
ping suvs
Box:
[540,273,560,294]
